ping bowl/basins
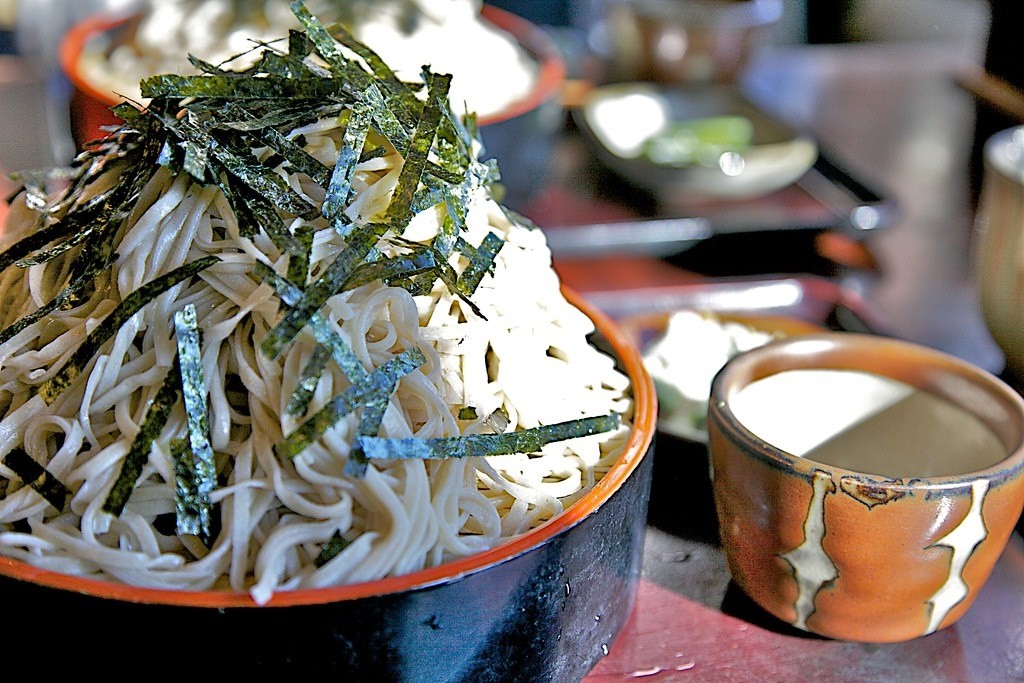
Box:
[60,4,567,211]
[0,284,660,683]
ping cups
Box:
[710,333,1024,644]
[971,124,1024,398]
[580,0,782,92]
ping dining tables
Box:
[0,1,1024,681]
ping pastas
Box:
[0,110,634,601]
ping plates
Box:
[577,83,818,199]
[615,311,836,447]
